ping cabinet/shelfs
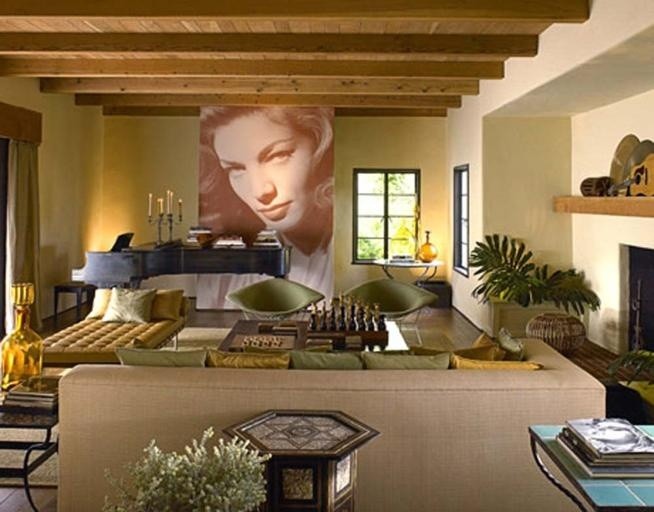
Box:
[139,241,293,289]
[489,296,581,339]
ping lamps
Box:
[148,188,183,246]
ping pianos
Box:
[69,225,178,317]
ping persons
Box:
[199,105,334,309]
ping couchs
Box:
[57,335,607,512]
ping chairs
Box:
[226,277,326,322]
[338,277,441,346]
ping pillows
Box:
[204,348,291,369]
[359,350,449,372]
[98,286,157,325]
[152,288,184,323]
[111,345,207,367]
[409,328,545,372]
[289,349,366,370]
[87,287,112,320]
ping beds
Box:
[43,296,197,364]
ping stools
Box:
[525,312,587,355]
[53,282,96,324]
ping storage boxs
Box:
[415,279,453,314]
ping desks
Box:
[372,257,445,288]
[527,420,654,511]
[0,389,58,511]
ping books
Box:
[556,416,653,478]
[390,255,414,263]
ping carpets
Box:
[217,318,310,353]
